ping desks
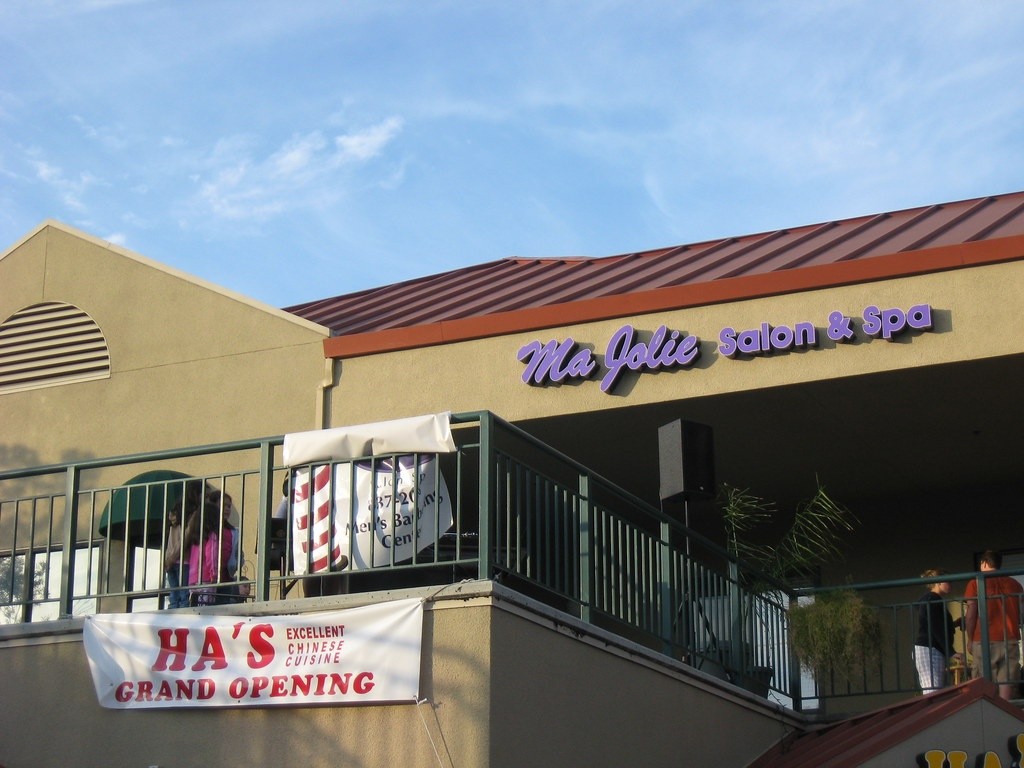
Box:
[946,665,971,685]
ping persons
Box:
[161,491,250,608]
[276,478,340,597]
[911,570,966,699]
[962,550,1024,700]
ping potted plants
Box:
[720,476,864,699]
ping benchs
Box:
[254,518,522,598]
